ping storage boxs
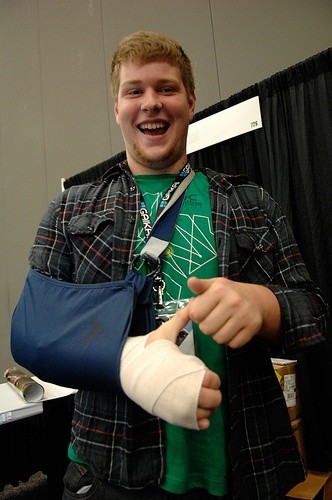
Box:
[270,356,300,421]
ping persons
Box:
[10,31,321,500]
